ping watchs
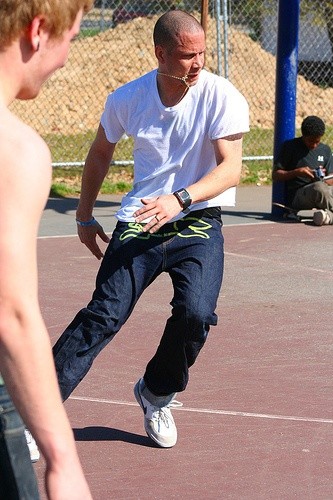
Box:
[174,188,191,212]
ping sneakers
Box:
[134,378,183,448]
[24,429,41,460]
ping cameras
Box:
[313,166,325,179]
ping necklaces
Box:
[156,71,190,106]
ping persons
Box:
[273,116,333,226]
[22,10,250,462]
[0,0,98,499]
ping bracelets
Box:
[75,218,96,226]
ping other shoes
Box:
[314,210,330,225]
